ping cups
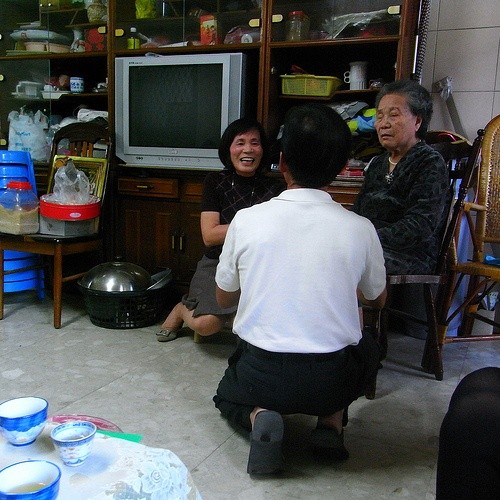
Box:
[70,77,84,93]
[343,61,369,91]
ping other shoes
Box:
[247,409,283,475]
[305,427,350,465]
[156,319,185,341]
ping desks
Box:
[0,423,202,500]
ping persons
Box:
[351,79,451,276]
[212,104,387,475]
[156,117,282,342]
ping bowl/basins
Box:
[79,264,173,291]
[0,396,49,446]
[0,461,61,500]
[24,42,70,53]
[52,421,96,467]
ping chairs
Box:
[0,122,113,329]
[363,114,500,400]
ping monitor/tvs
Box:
[114,52,248,169]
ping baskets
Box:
[78,282,174,330]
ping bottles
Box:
[0,181,39,235]
[70,30,85,52]
[127,28,140,51]
[285,10,308,40]
[86,0,107,23]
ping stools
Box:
[0,151,46,299]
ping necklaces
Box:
[388,157,397,165]
[231,170,255,212]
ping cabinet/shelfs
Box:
[263,0,422,212]
[111,0,267,306]
[0,0,117,294]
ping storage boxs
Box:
[40,210,90,236]
[279,74,342,96]
[201,15,225,44]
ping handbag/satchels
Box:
[52,116,110,146]
[8,109,52,162]
